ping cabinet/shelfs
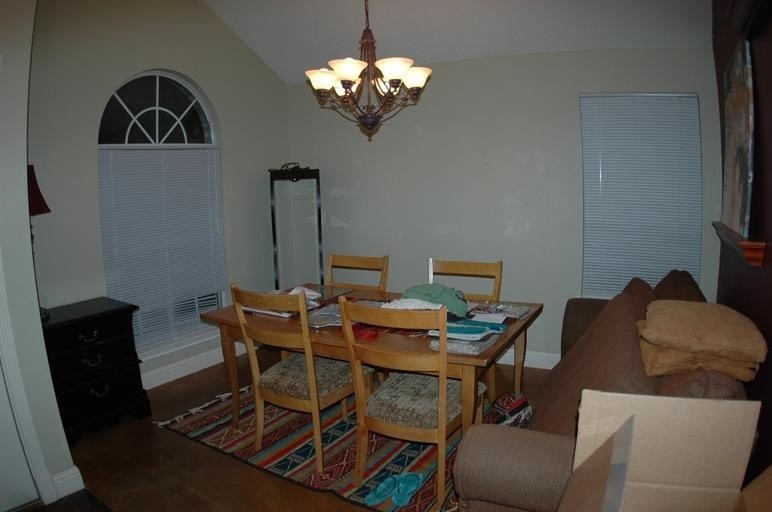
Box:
[40,298,151,434]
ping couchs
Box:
[450,267,772,511]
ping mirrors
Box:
[268,165,327,289]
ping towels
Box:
[253,287,322,315]
[436,323,502,356]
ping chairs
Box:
[229,289,379,472]
[326,254,391,298]
[336,295,486,510]
[421,257,504,401]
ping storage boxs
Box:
[559,387,771,510]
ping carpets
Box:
[155,379,534,511]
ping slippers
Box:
[365,472,423,506]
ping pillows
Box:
[402,284,471,315]
[636,300,763,381]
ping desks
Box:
[202,283,545,431]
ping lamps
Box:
[308,1,435,143]
[26,162,55,323]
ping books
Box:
[429,333,502,356]
[476,299,533,320]
[428,318,508,342]
